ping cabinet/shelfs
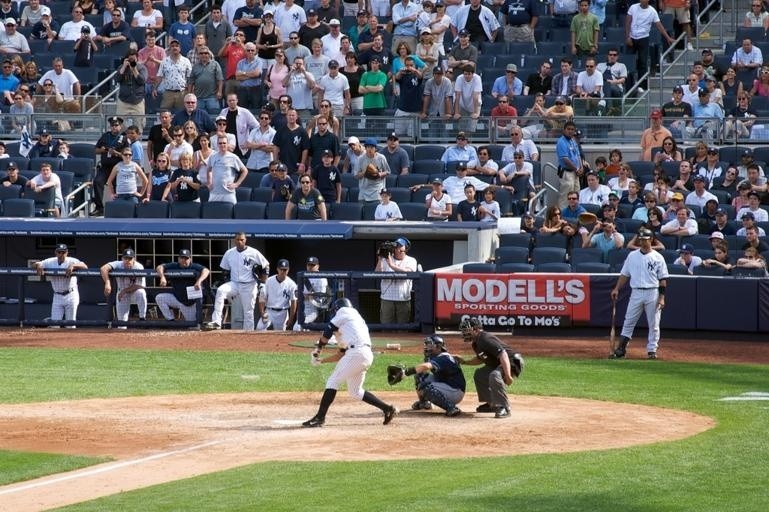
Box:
[115,236,232,289]
[112,302,233,325]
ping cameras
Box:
[296,63,302,68]
[129,58,137,67]
[231,37,237,42]
[84,34,88,38]
[600,224,609,228]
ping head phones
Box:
[402,236,411,249]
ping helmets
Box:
[253,263,266,283]
[637,229,654,240]
[461,318,483,342]
[424,335,447,356]
[334,298,353,311]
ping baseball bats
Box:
[609,299,616,357]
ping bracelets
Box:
[658,285,666,295]
[319,338,327,348]
[404,367,416,375]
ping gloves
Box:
[311,348,322,365]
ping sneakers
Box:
[446,407,460,416]
[496,407,511,417]
[413,402,431,409]
[615,347,625,357]
[648,351,656,359]
[201,321,218,330]
[383,405,396,425]
[477,403,501,412]
[303,415,325,425]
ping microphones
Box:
[400,248,410,252]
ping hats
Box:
[394,238,403,246]
[307,257,318,264]
[522,210,536,220]
[380,188,390,195]
[180,250,190,257]
[55,243,67,252]
[123,249,135,257]
[278,260,289,269]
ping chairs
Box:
[0,0,768,278]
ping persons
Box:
[454,312,523,417]
[301,297,396,425]
[386,335,466,416]
[374,236,417,325]
[606,226,669,358]
[30,232,328,333]
[0,1,769,275]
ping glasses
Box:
[0,0,546,184]
[552,53,769,254]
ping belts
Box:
[351,344,370,348]
[56,288,73,296]
[269,308,283,311]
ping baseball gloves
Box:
[387,365,403,386]
[578,211,598,225]
[365,163,379,179]
